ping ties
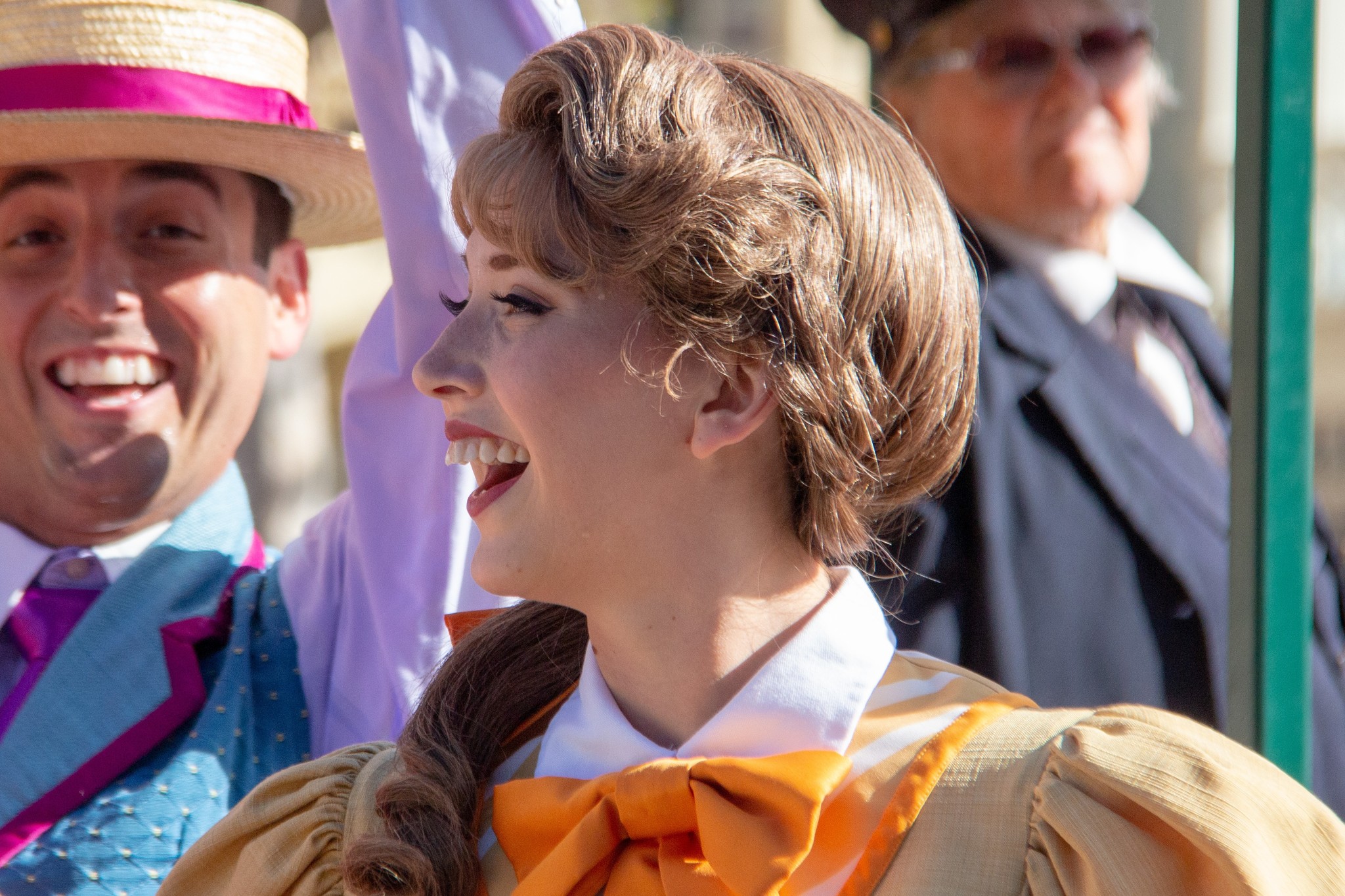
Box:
[0,587,108,741]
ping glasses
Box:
[892,15,1152,90]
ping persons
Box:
[824,0,1345,819]
[0,0,593,896]
[157,23,1344,896]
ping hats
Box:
[2,1,384,251]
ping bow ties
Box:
[489,749,851,896]
